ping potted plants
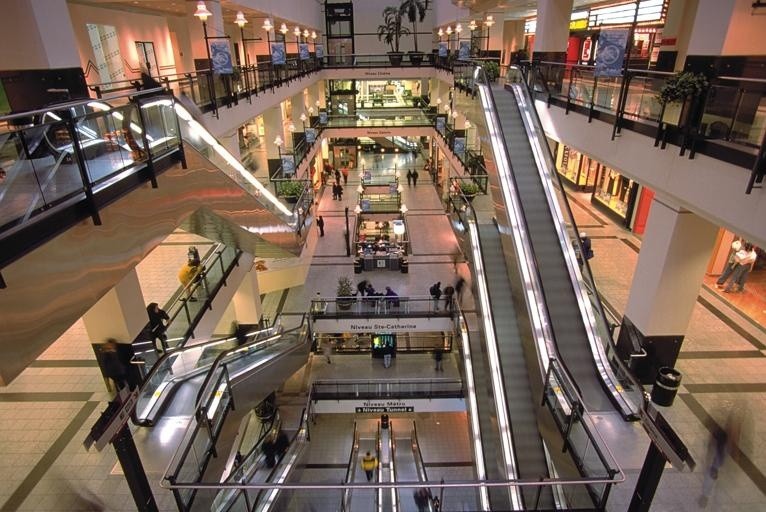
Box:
[282,180,305,203]
[459,182,479,203]
[378,0,430,66]
[334,277,353,310]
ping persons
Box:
[273,429,290,458]
[455,276,465,302]
[363,144,372,162]
[336,182,343,201]
[326,163,332,176]
[433,344,445,372]
[580,231,592,262]
[325,161,331,169]
[234,450,241,464]
[411,149,417,161]
[358,280,368,296]
[347,153,355,169]
[518,50,529,67]
[176,258,202,302]
[364,284,375,296]
[140,73,162,92]
[322,338,333,364]
[411,169,419,186]
[384,286,398,303]
[186,246,201,265]
[99,337,130,400]
[382,340,393,368]
[433,281,442,311]
[359,451,378,481]
[694,428,730,508]
[718,238,747,286]
[412,487,430,511]
[720,242,758,295]
[244,150,259,172]
[261,432,276,468]
[335,169,341,186]
[316,215,325,237]
[432,495,441,511]
[342,166,349,184]
[407,170,412,187]
[144,302,173,355]
[443,281,454,310]
[332,182,337,200]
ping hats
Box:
[580,232,586,237]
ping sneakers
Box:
[716,281,744,294]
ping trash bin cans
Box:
[381,415,388,428]
[651,369,681,405]
